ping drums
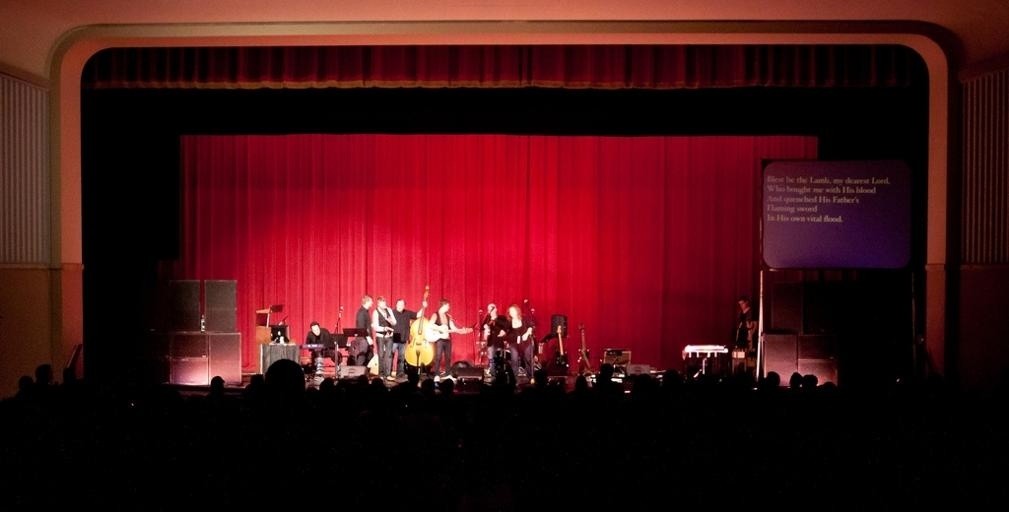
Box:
[479,347,512,375]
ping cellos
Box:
[405,283,437,367]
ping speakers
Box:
[624,364,650,377]
[339,366,370,381]
[760,268,839,387]
[535,366,568,386]
[457,367,484,383]
[169,280,242,385]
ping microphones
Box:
[524,300,530,307]
[490,306,495,313]
[531,309,535,315]
[479,310,483,315]
[339,305,344,312]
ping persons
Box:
[497,303,536,375]
[305,321,342,369]
[479,302,508,376]
[733,295,759,350]
[431,300,467,381]
[1,359,1009,511]
[356,294,374,366]
[391,298,429,379]
[371,297,396,382]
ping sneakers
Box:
[387,376,395,381]
[433,375,440,382]
[446,375,457,381]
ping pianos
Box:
[300,343,352,350]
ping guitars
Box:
[577,322,591,373]
[554,325,569,372]
[425,324,474,342]
